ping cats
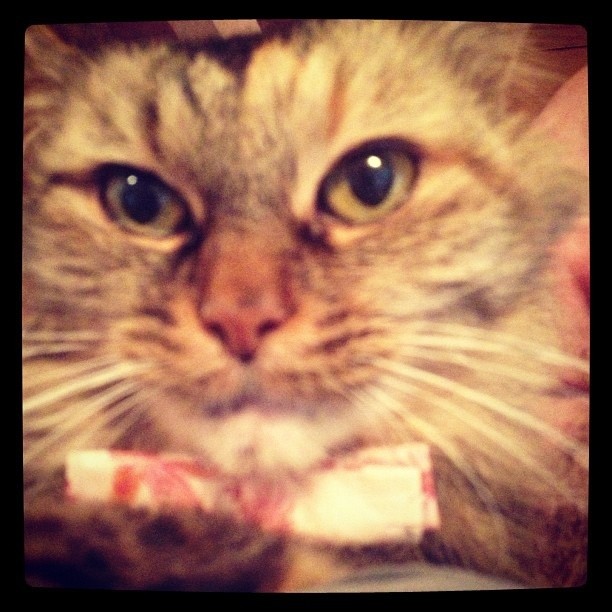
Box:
[21,17,589,589]
